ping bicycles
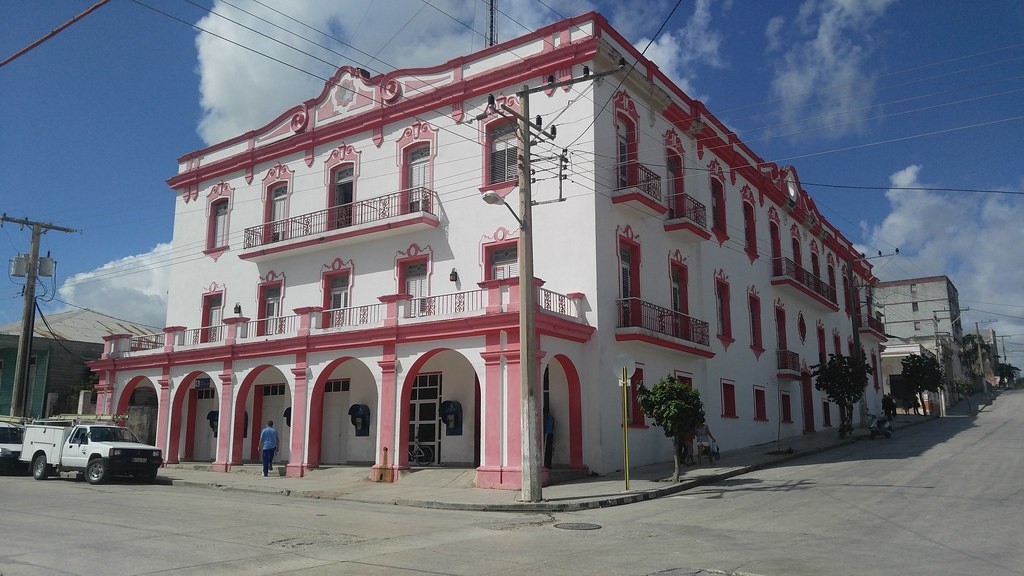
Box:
[407,425,435,466]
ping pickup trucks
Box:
[16,411,164,486]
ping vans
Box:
[0,420,25,477]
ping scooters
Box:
[865,407,893,441]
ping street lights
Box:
[479,190,542,504]
[851,301,887,430]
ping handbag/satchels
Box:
[715,446,720,461]
[712,440,717,453]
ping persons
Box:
[678,417,717,466]
[913,394,921,415]
[903,398,910,415]
[543,408,554,468]
[258,421,279,476]
[882,393,897,421]
